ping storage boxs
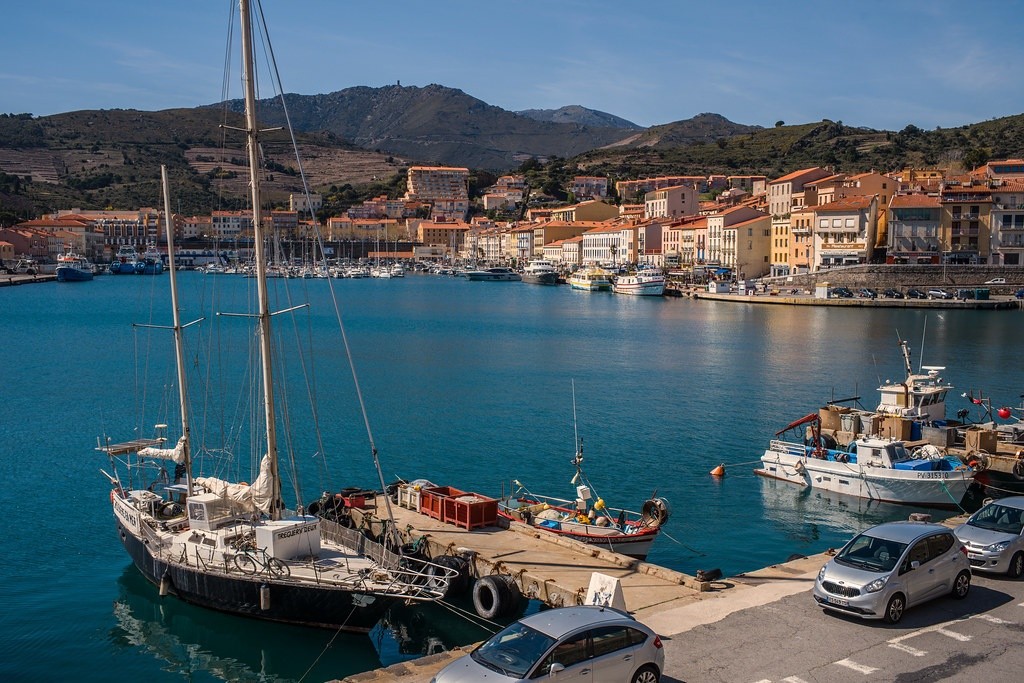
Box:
[398,484,434,513]
[922,425,956,446]
[839,411,866,433]
[420,486,467,522]
[859,414,879,435]
[879,414,912,441]
[966,429,997,455]
[443,492,499,531]
[819,406,850,431]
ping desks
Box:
[128,489,162,518]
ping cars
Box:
[12,264,38,275]
[812,520,972,626]
[833,287,854,297]
[927,289,953,299]
[907,289,926,299]
[955,289,975,299]
[953,496,1023,578]
[859,288,878,298]
[429,605,665,683]
[883,288,904,299]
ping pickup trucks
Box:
[985,278,1005,284]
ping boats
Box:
[566,260,613,291]
[94,0,461,640]
[521,258,560,286]
[461,267,522,281]
[92,217,490,278]
[55,251,94,282]
[497,377,672,561]
[874,311,1024,499]
[611,264,666,296]
[753,381,977,510]
